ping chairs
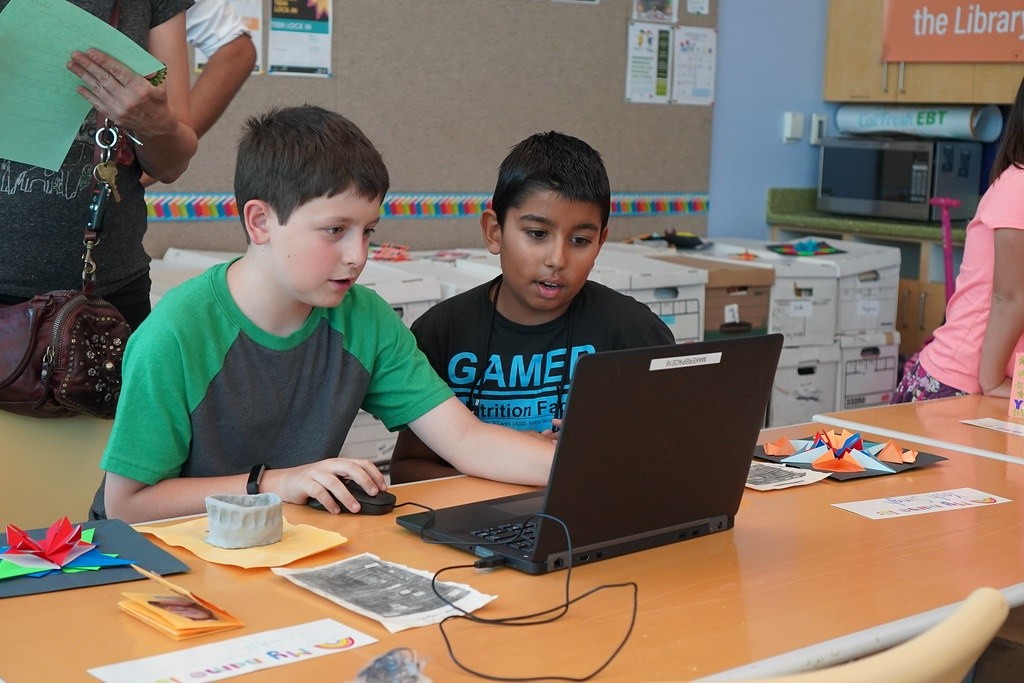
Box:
[717,586,1009,683]
[0,408,114,534]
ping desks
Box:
[813,395,1024,465]
[0,425,1024,683]
[896,276,948,360]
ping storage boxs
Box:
[150,237,901,427]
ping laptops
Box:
[396,332,785,576]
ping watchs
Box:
[247,463,270,495]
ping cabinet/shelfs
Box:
[822,0,1024,104]
[770,225,965,282]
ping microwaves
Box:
[816,138,984,223]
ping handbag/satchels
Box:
[0,287,133,416]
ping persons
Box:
[149,601,214,620]
[890,78,1024,403]
[390,131,675,484]
[89,104,556,523]
[0,0,197,332]
[140,0,257,188]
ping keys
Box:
[97,162,121,202]
[120,126,143,146]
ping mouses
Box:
[307,478,397,516]
